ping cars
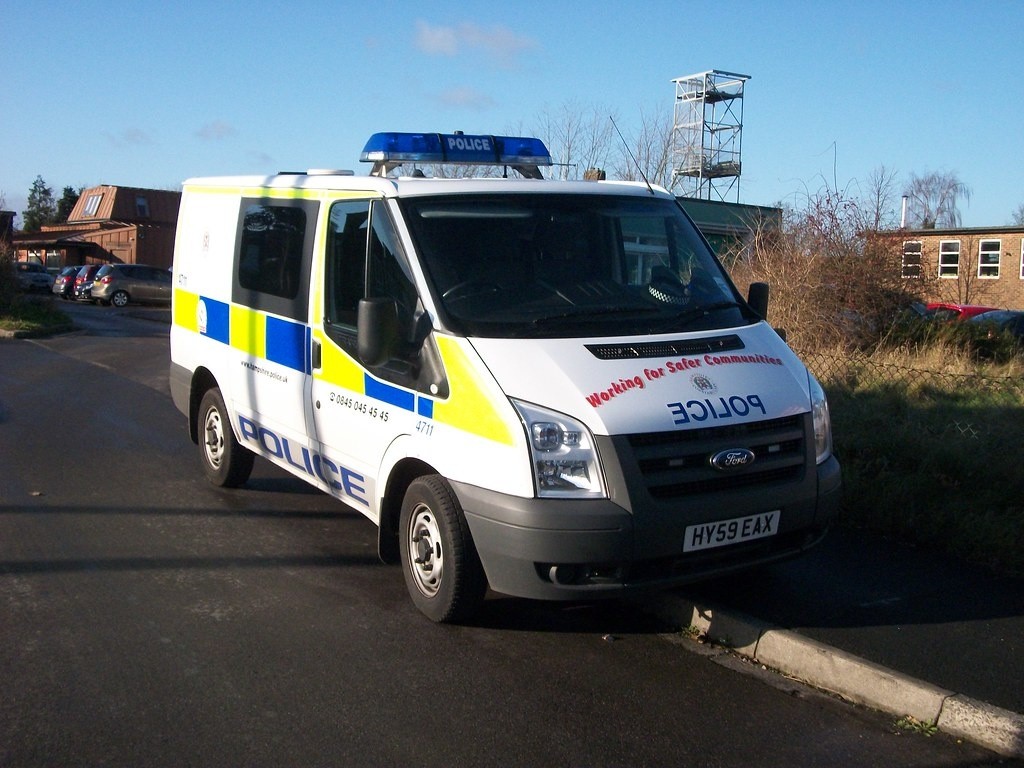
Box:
[15,261,55,292]
[970,310,1024,342]
[927,303,999,322]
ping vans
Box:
[89,263,172,308]
[74,265,111,306]
[168,131,849,626]
[51,265,81,301]
